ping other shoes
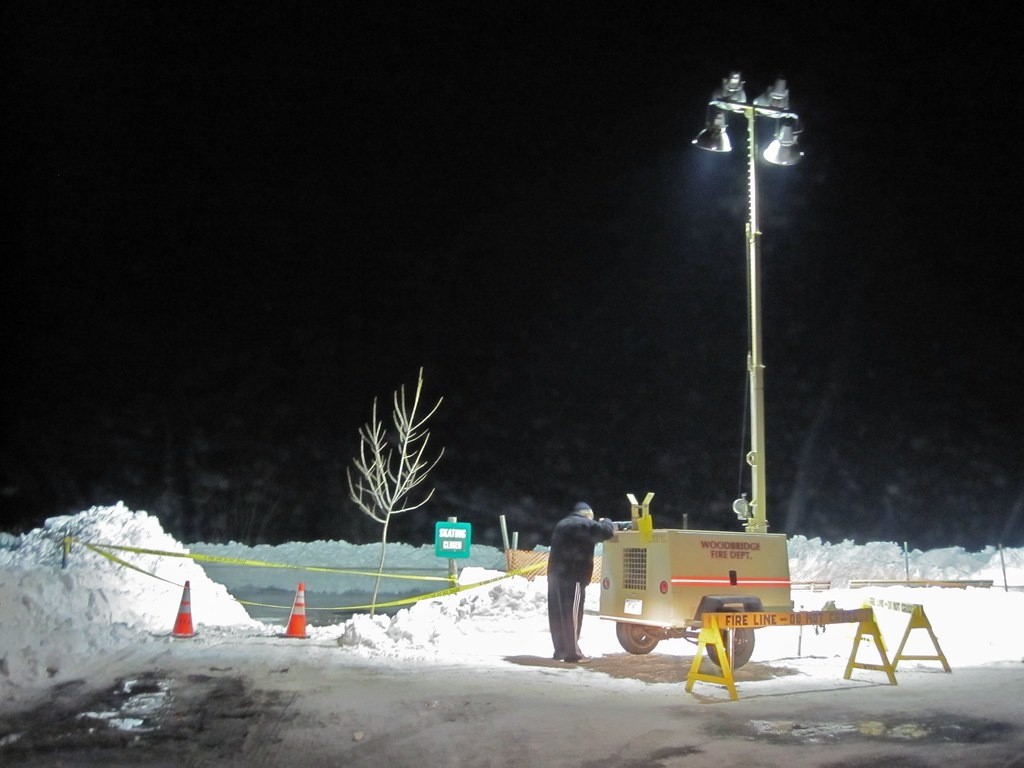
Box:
[554,654,566,661]
[576,654,592,664]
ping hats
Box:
[574,502,592,512]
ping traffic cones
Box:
[169,580,200,638]
[276,582,311,639]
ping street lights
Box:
[690,65,807,534]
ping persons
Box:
[546,502,614,663]
